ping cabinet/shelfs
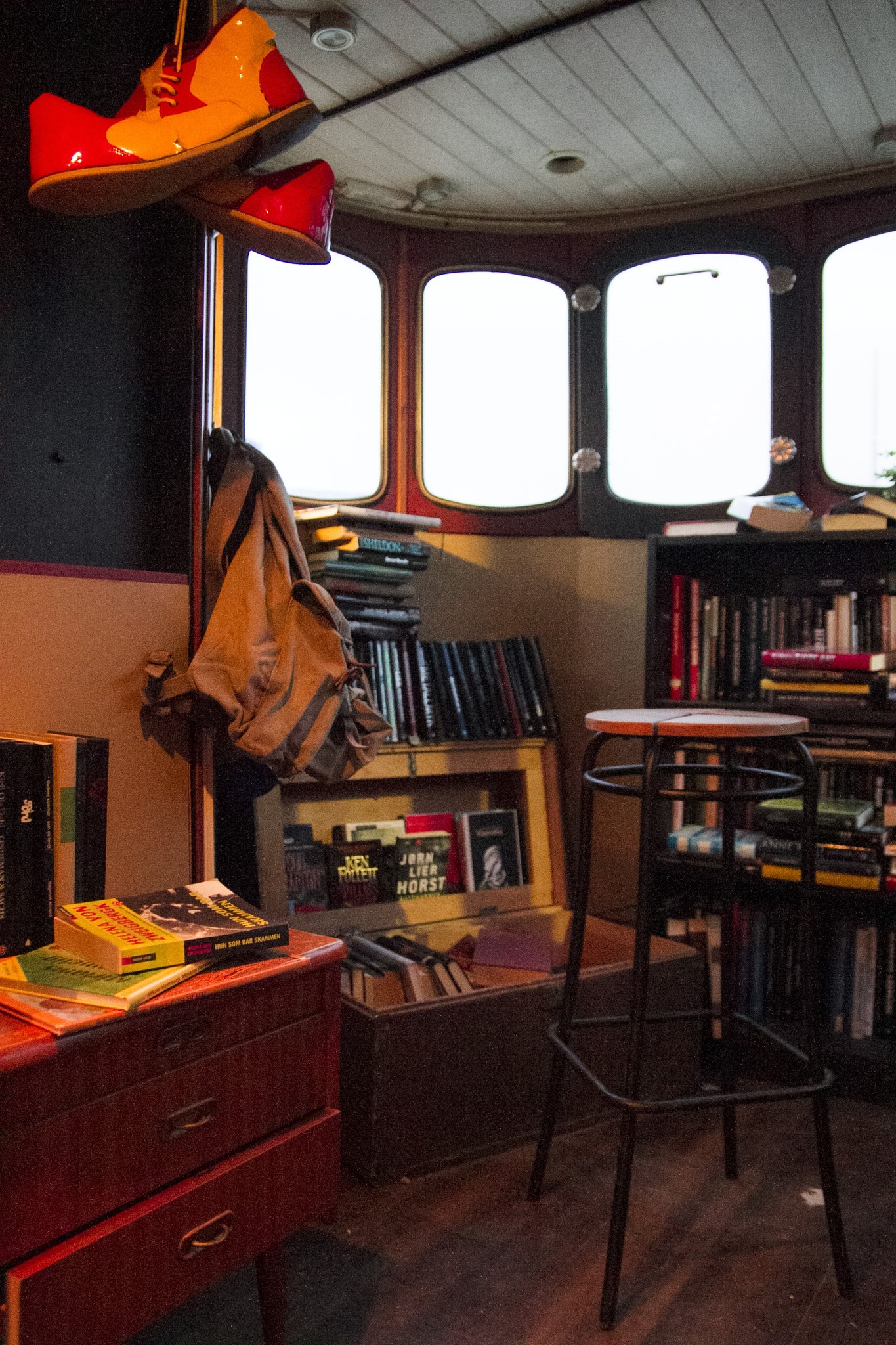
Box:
[0,925,348,1345]
[640,531,896,1108]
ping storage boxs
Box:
[249,736,702,1190]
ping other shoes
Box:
[29,2,323,217]
[173,158,335,265]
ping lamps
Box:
[309,9,358,51]
[417,178,453,204]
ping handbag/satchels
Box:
[139,426,393,787]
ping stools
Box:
[526,708,857,1328]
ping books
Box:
[0,727,289,1037]
[331,924,552,1012]
[292,504,562,743]
[670,796,896,890]
[666,894,896,1051]
[281,809,522,913]
[663,491,896,537]
[670,574,896,713]
